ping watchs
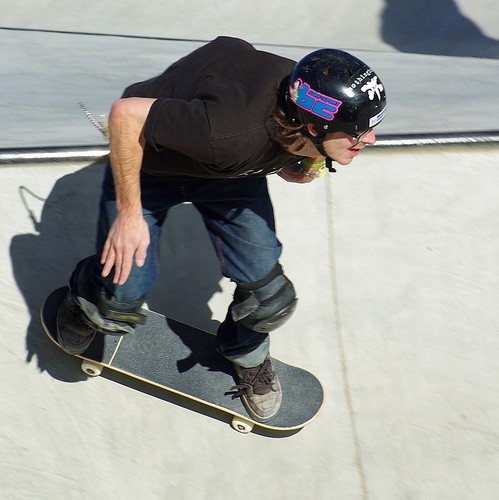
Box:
[297,165,328,178]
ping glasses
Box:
[340,128,374,142]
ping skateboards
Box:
[40,283,326,434]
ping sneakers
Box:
[230,353,282,424]
[56,300,97,355]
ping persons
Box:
[56,35,386,423]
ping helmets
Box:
[287,48,387,174]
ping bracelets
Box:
[301,160,325,168]
